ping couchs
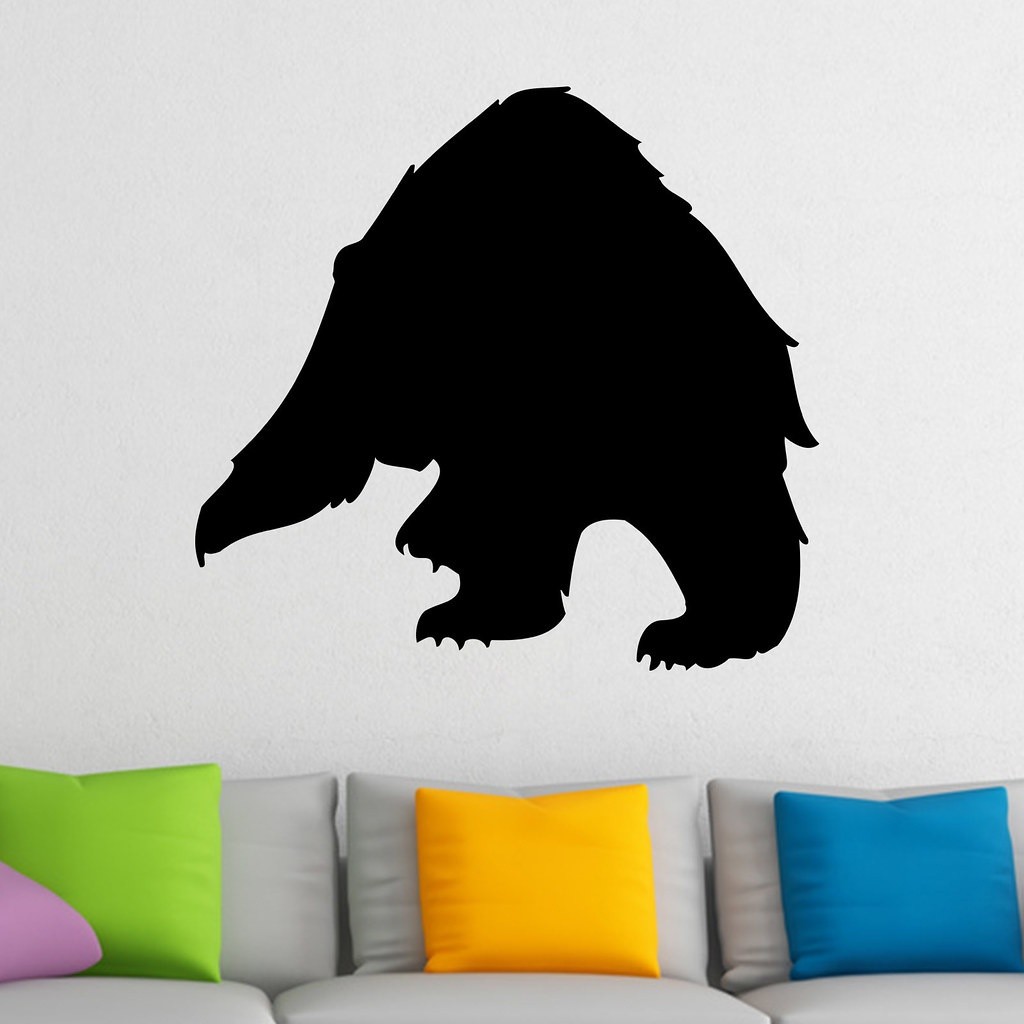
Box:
[0,771,1024,1023]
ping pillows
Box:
[772,786,1024,982]
[1,862,103,982]
[414,784,662,979]
[1,766,222,983]
